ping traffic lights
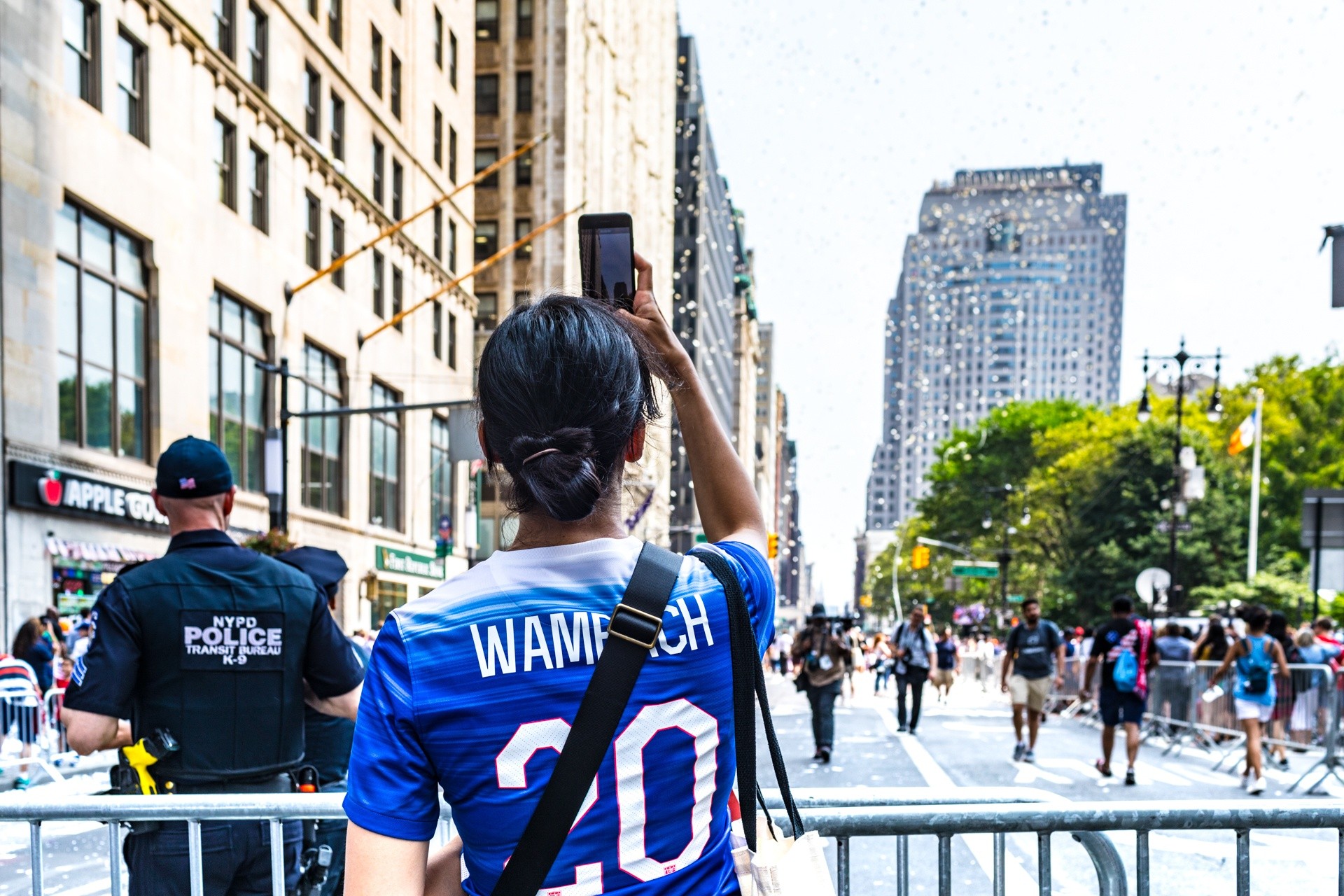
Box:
[922,546,930,568]
[768,533,779,559]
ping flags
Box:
[1228,408,1258,456]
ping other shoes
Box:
[1239,776,1267,794]
[1095,758,1113,777]
[1014,743,1025,760]
[1277,758,1288,771]
[1125,770,1136,786]
[813,747,830,761]
[1025,750,1034,763]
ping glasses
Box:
[912,612,923,618]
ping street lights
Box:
[1138,336,1232,605]
[982,482,1031,628]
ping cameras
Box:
[807,657,819,671]
[813,617,827,631]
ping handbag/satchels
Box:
[686,551,834,896]
[793,669,812,693]
[1113,649,1138,692]
[1293,658,1312,692]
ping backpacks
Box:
[1243,665,1268,695]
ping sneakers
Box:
[14,773,30,791]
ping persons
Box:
[1002,598,1066,764]
[921,604,1020,705]
[829,617,898,702]
[57,435,366,896]
[0,605,99,792]
[241,531,386,896]
[1041,622,1128,729]
[340,251,771,896]
[1206,606,1293,796]
[1134,602,1344,772]
[1079,598,1162,786]
[793,604,854,764]
[758,620,802,682]
[888,604,937,734]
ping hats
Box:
[274,545,349,595]
[154,436,233,499]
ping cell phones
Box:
[578,212,635,314]
